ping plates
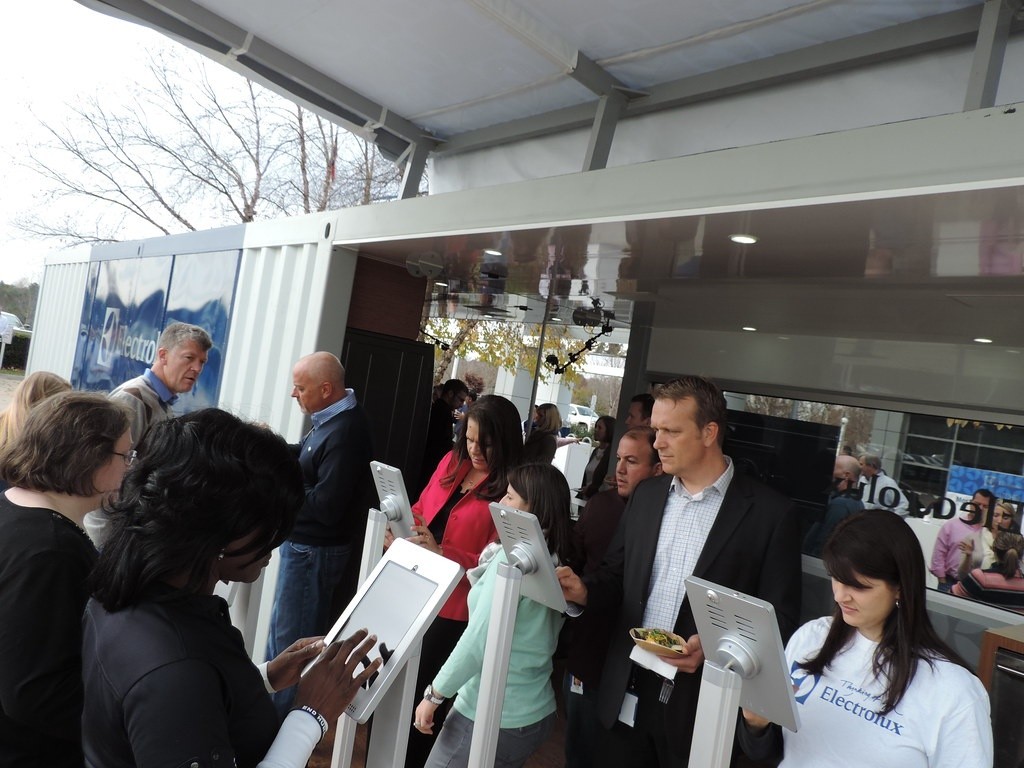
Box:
[630,627,687,658]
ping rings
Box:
[413,722,420,726]
[425,534,430,542]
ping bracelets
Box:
[287,706,326,741]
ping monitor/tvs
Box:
[489,501,569,613]
[298,536,465,725]
[370,461,420,546]
[683,576,803,734]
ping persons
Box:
[553,375,801,768]
[421,379,615,515]
[927,489,1024,596]
[80,409,382,768]
[801,447,909,559]
[382,395,575,768]
[742,509,993,768]
[563,393,666,768]
[83,322,212,552]
[262,352,374,705]
[0,370,137,768]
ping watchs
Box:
[424,685,445,705]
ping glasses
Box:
[112,450,138,466]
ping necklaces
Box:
[466,475,482,486]
